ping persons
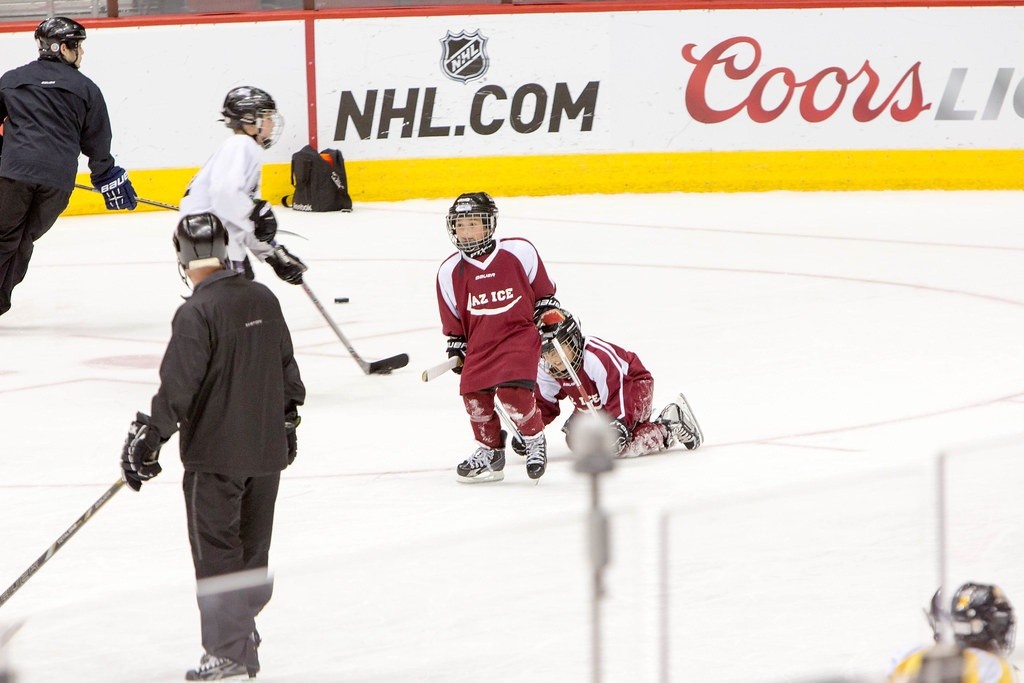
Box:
[436,191,557,486]
[891,583,1018,683]
[511,308,703,459]
[179,85,307,302]
[119,212,307,683]
[0,16,137,315]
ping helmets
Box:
[173,213,229,268]
[446,192,498,252]
[932,581,1012,646]
[222,86,283,147]
[538,308,583,378]
[35,17,86,53]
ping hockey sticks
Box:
[550,335,598,417]
[74,182,308,240]
[0,471,127,607]
[422,349,523,443]
[300,278,409,374]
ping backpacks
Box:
[282,145,352,211]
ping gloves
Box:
[265,245,306,285]
[446,336,468,373]
[96,168,137,210]
[249,201,276,243]
[121,412,161,491]
[610,418,630,458]
[533,297,560,324]
[286,416,301,460]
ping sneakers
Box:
[524,431,546,483]
[655,394,702,451]
[457,430,507,482]
[512,428,526,455]
[186,641,255,683]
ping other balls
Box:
[334,298,349,303]
